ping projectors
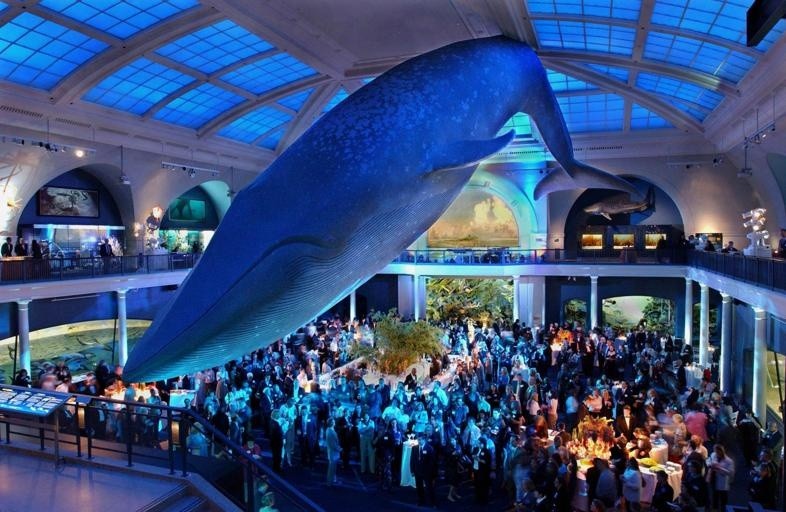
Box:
[119,176,131,184]
[736,168,752,178]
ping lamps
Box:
[147,206,163,234]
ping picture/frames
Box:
[37,184,101,219]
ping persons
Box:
[1,224,785,512]
